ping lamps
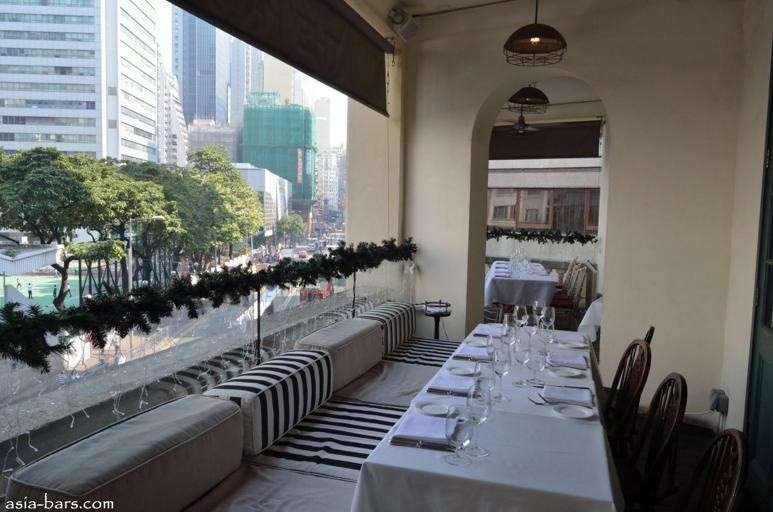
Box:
[501,81,549,135]
[503,0,567,66]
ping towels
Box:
[542,330,595,405]
[453,342,495,362]
[392,413,459,450]
[473,322,505,339]
[427,372,478,397]
[495,261,508,277]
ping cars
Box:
[299,251,306,258]
[308,244,315,253]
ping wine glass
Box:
[510,246,527,274]
[445,301,555,467]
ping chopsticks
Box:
[390,437,455,454]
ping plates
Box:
[553,341,594,422]
[415,339,492,416]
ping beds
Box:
[485,260,553,322]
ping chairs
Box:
[553,257,588,330]
[607,326,748,511]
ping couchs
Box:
[5,302,463,512]
